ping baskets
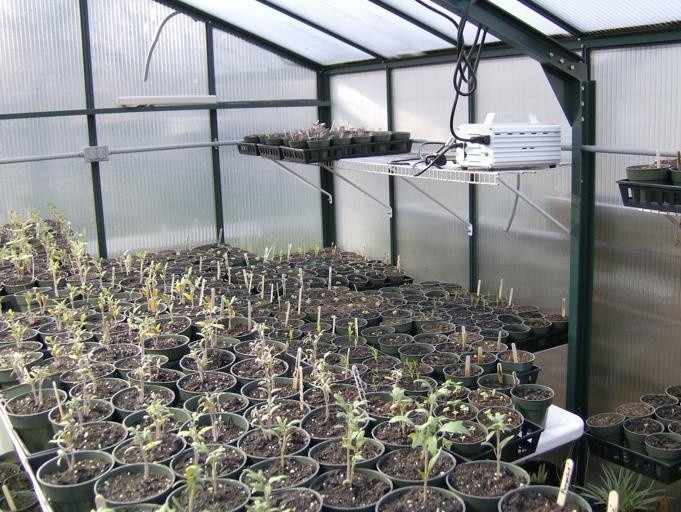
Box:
[506,326,567,354]
[236,141,258,156]
[585,431,665,486]
[257,144,283,161]
[616,178,681,213]
[447,417,544,466]
[350,275,414,292]
[280,139,415,165]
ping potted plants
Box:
[242,119,411,159]
[624,147,681,204]
